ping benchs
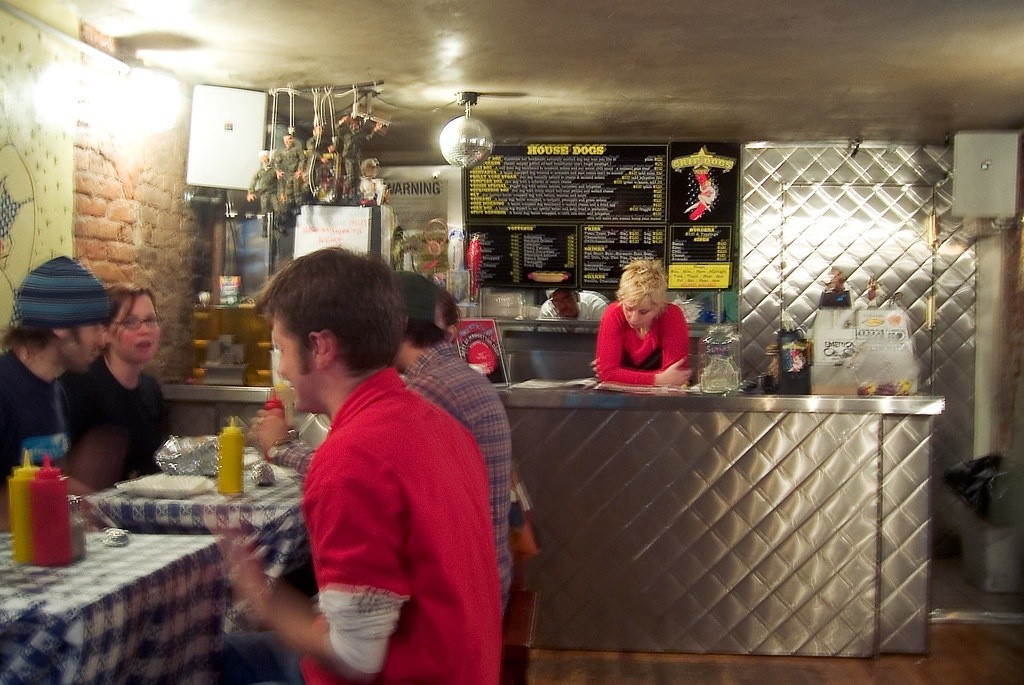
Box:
[500,580,537,685]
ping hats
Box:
[15,255,111,329]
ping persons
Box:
[591,259,692,386]
[536,290,609,322]
[1,246,520,685]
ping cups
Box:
[198,291,210,311]
[267,389,297,431]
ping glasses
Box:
[113,315,162,330]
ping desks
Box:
[79,447,321,640]
[0,533,227,685]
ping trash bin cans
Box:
[942,452,1024,594]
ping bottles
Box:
[698,324,742,395]
[217,417,245,494]
[30,456,73,568]
[8,450,40,564]
[67,494,87,562]
